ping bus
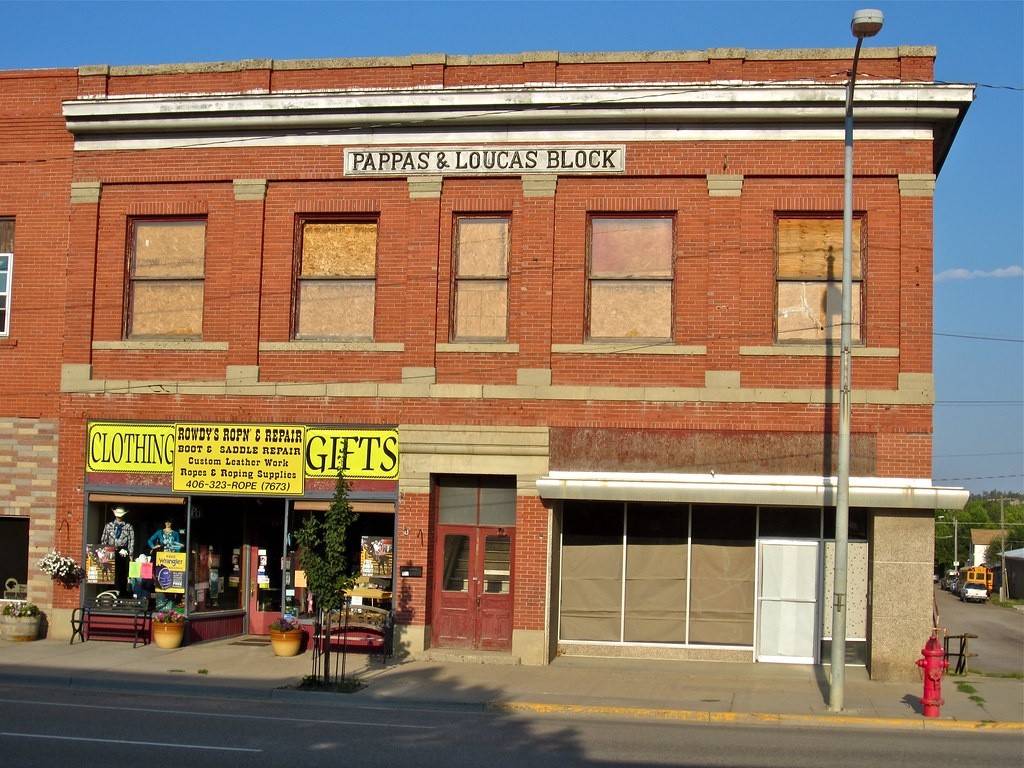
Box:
[955,565,994,597]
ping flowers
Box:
[1,602,46,618]
[35,550,84,587]
[152,606,189,624]
[267,616,304,633]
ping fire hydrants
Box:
[915,635,951,718]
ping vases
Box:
[0,615,42,643]
[154,623,186,649]
[270,631,302,658]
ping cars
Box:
[959,581,988,604]
[933,569,959,595]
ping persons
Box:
[101,507,134,595]
[148,517,180,552]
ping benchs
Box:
[69,597,157,648]
[311,604,393,659]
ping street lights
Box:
[829,7,884,714]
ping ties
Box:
[116,524,124,539]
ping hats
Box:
[112,507,129,517]
[136,554,152,563]
